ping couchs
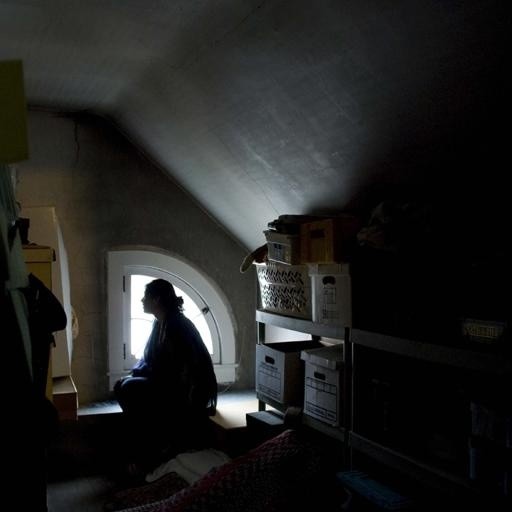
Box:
[102,429,342,511]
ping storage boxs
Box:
[266,215,355,265]
[50,376,78,422]
[311,263,357,325]
[255,340,343,427]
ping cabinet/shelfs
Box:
[252,310,512,512]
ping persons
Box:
[113,279,217,415]
